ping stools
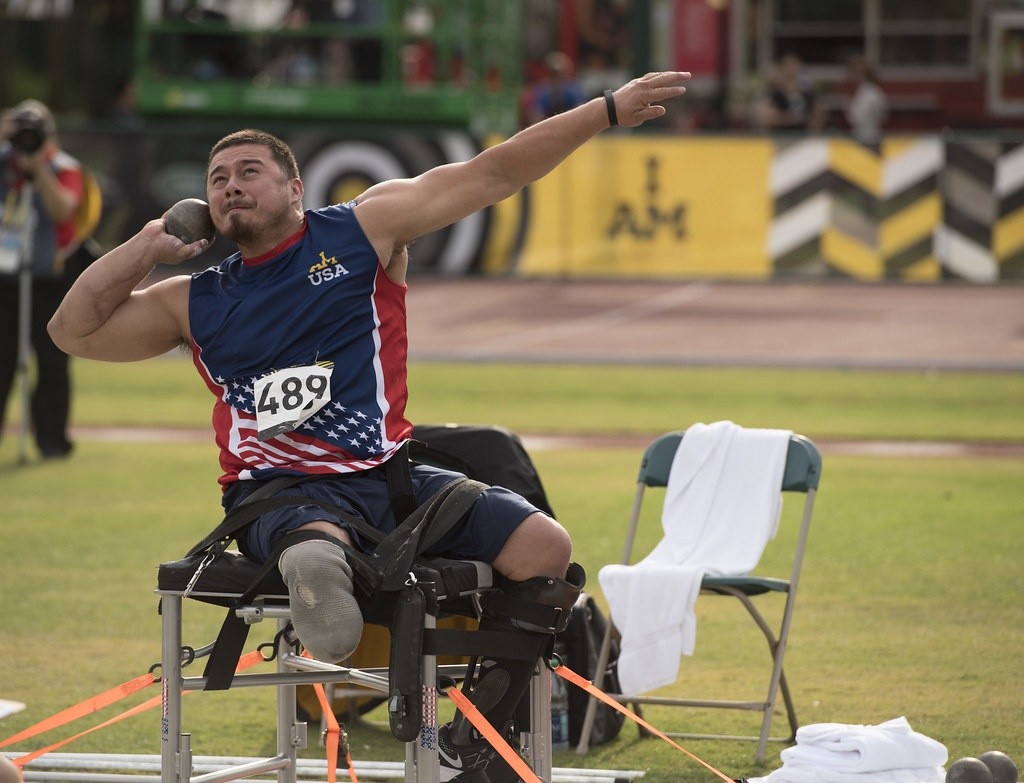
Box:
[157,551,553,783]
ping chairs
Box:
[578,421,822,767]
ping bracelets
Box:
[600,90,620,128]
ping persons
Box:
[46,63,699,783]
[2,98,99,467]
[520,45,891,138]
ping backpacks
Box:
[564,592,630,745]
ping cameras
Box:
[9,108,46,152]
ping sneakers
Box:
[437,723,525,782]
